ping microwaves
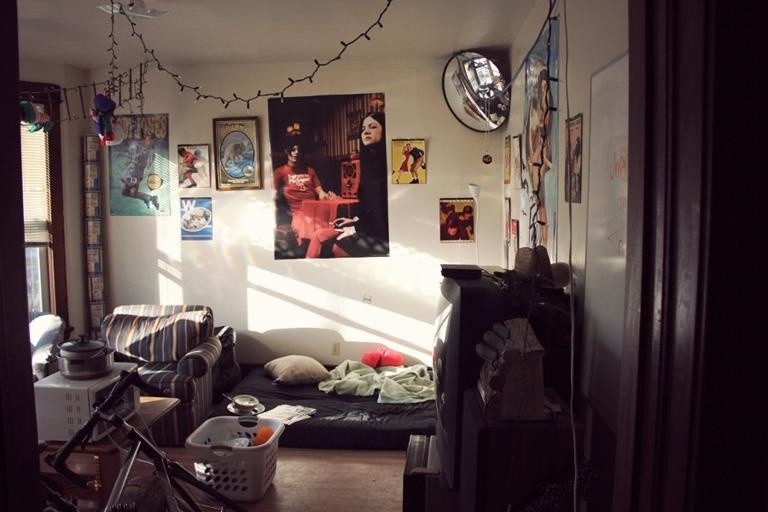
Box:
[34,362,140,444]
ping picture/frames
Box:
[212,116,262,192]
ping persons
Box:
[112,134,164,210]
[524,69,551,248]
[274,141,332,247]
[178,147,200,189]
[306,110,388,258]
[392,145,414,184]
[439,202,474,240]
[406,144,426,184]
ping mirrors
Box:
[442,51,510,132]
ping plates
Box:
[181,207,212,232]
[226,402,266,415]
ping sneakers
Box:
[144,195,152,211]
[152,194,160,210]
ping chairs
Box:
[97,304,222,447]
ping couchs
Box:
[28,311,65,383]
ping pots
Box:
[57,334,106,358]
[55,348,116,380]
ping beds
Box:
[204,364,436,451]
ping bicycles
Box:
[45,370,248,512]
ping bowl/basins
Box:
[233,394,259,413]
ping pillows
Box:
[101,304,214,364]
[264,355,331,385]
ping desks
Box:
[38,397,181,512]
[38,443,406,512]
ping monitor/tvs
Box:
[434,275,569,490]
